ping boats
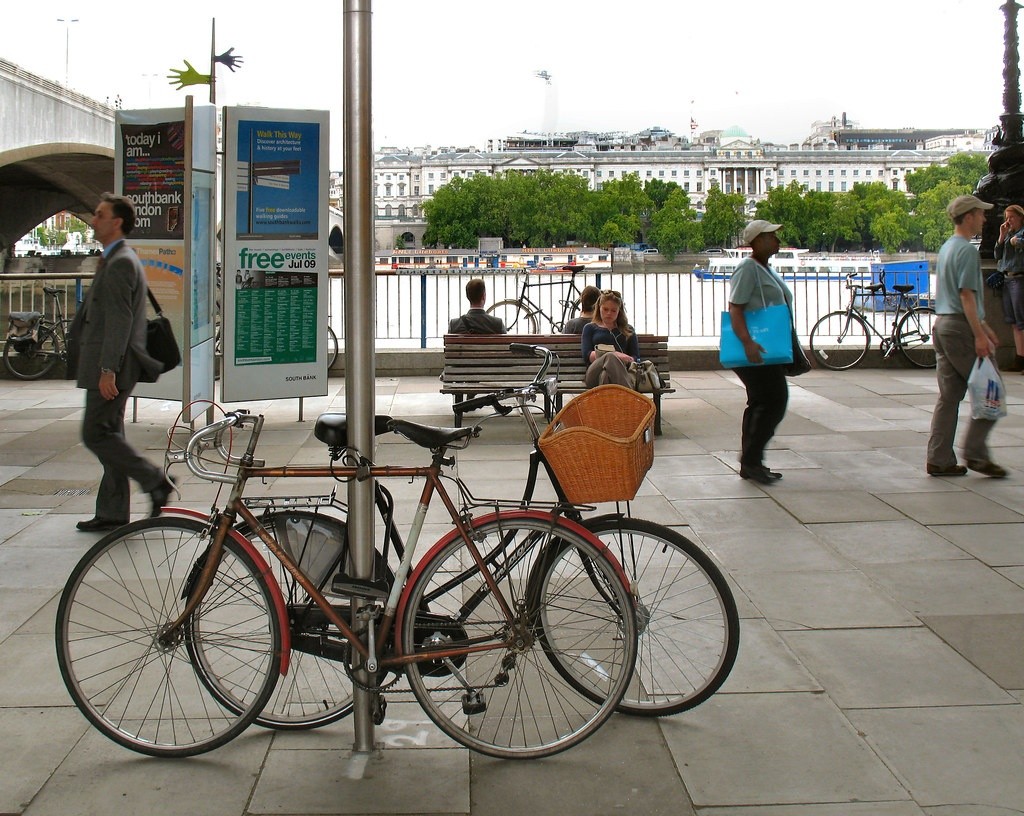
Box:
[690,248,882,283]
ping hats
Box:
[742,220,783,243]
[945,195,994,218]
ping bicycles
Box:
[810,272,938,370]
[486,266,604,334]
[179,342,739,731]
[55,400,638,759]
[3,287,85,381]
[214,299,338,383]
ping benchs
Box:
[439,335,675,441]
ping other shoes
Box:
[964,458,1007,479]
[739,463,782,483]
[150,475,175,519]
[1003,355,1024,372]
[927,464,966,477]
[76,515,130,531]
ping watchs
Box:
[101,367,115,374]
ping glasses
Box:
[601,289,621,299]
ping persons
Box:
[561,285,601,334]
[66,192,181,532]
[447,279,507,399]
[994,205,1024,374]
[729,220,793,483]
[581,290,639,390]
[927,194,1007,478]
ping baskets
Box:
[538,384,656,504]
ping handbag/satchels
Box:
[628,360,662,391]
[144,318,182,376]
[778,331,811,376]
[968,355,1007,420]
[719,304,793,370]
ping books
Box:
[594,344,615,359]
